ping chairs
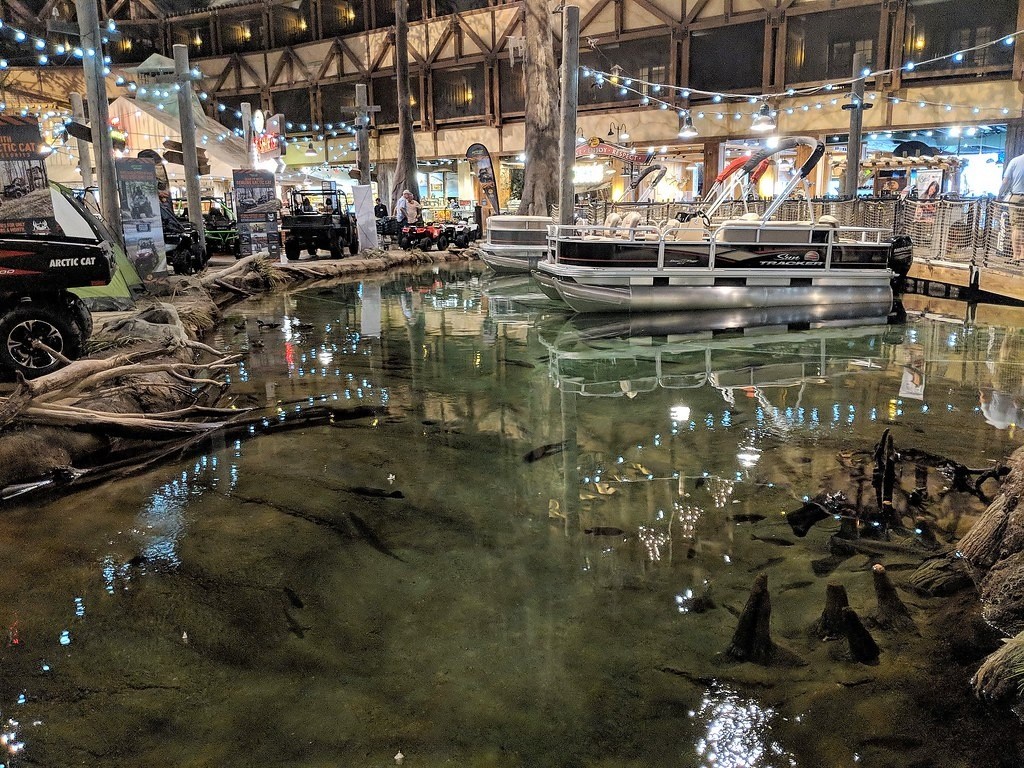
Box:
[644,219,679,240]
[598,213,640,242]
[582,213,620,240]
[819,215,857,243]
[207,207,221,217]
[715,213,759,242]
[180,207,189,217]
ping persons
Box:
[393,190,422,243]
[918,181,939,217]
[996,154,1024,266]
[374,198,388,218]
[451,199,455,209]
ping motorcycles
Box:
[442,214,481,249]
[401,222,447,252]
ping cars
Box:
[238,193,268,210]
[3,178,30,200]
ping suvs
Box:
[280,189,359,260]
[177,196,241,259]
[130,196,153,220]
[156,199,207,276]
[135,238,159,270]
[0,115,116,380]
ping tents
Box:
[49,178,144,312]
[45,96,378,213]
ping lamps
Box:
[304,142,317,157]
[619,123,629,139]
[576,127,585,143]
[607,122,617,136]
[749,98,776,131]
[677,112,698,137]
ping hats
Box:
[403,190,412,194]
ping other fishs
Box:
[602,586,642,590]
[584,527,622,535]
[898,580,932,597]
[884,563,919,569]
[723,604,741,619]
[537,354,549,359]
[694,476,707,491]
[905,601,928,610]
[498,358,534,368]
[851,556,875,569]
[738,361,766,370]
[283,609,303,638]
[906,421,923,434]
[521,439,572,460]
[847,543,884,557]
[726,513,766,522]
[779,580,816,594]
[881,418,902,427]
[748,556,790,572]
[860,734,923,751]
[350,512,404,562]
[499,334,527,346]
[751,532,792,547]
[727,410,745,415]
[282,584,303,608]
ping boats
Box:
[539,310,907,436]
[475,153,770,303]
[538,135,913,316]
